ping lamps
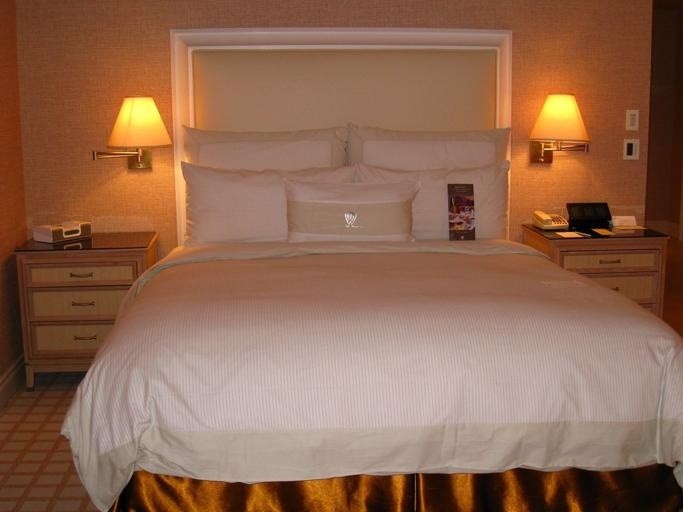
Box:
[528,94,593,164]
[93,96,173,170]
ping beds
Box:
[59,27,683,512]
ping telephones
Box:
[533,211,570,231]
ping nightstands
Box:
[520,223,672,319]
[13,231,160,392]
